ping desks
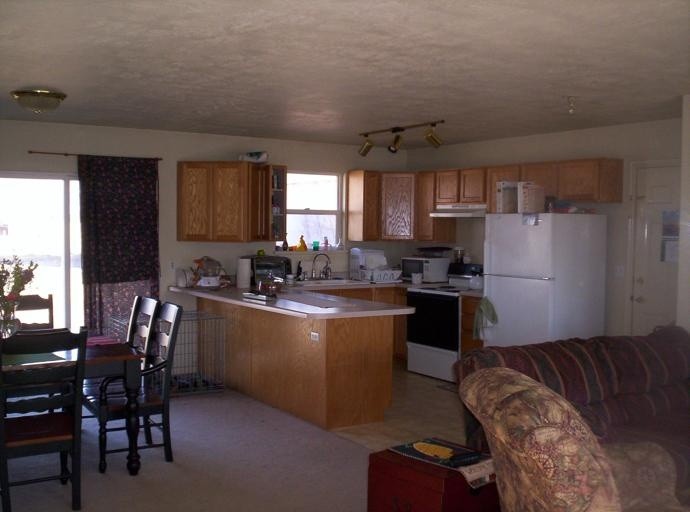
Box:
[0,335,149,477]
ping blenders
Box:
[196,257,222,290]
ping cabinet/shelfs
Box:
[381,171,456,243]
[347,170,380,241]
[312,289,373,300]
[520,157,624,204]
[435,167,486,203]
[176,160,249,244]
[373,288,396,357]
[249,162,287,242]
[486,165,520,214]
[366,436,500,512]
[461,296,483,353]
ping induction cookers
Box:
[407,286,474,296]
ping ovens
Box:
[241,255,292,287]
[408,291,460,383]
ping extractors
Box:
[429,204,486,218]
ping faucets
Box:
[311,253,331,279]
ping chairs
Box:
[82,302,185,474]
[0,326,89,512]
[79,296,161,398]
[0,295,55,418]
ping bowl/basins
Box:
[285,274,298,280]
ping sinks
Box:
[314,277,344,281]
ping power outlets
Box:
[310,332,319,341]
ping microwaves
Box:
[400,258,451,283]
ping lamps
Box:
[423,128,443,149]
[10,88,66,115]
[358,140,373,157]
[388,134,404,153]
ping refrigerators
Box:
[483,213,607,348]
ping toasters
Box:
[176,267,195,288]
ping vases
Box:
[0,298,21,339]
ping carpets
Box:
[0,389,374,511]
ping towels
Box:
[472,297,497,340]
[478,311,492,340]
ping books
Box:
[390,436,497,489]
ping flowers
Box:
[0,254,38,335]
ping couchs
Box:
[451,327,690,511]
[456,367,690,512]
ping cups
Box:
[312,239,321,251]
